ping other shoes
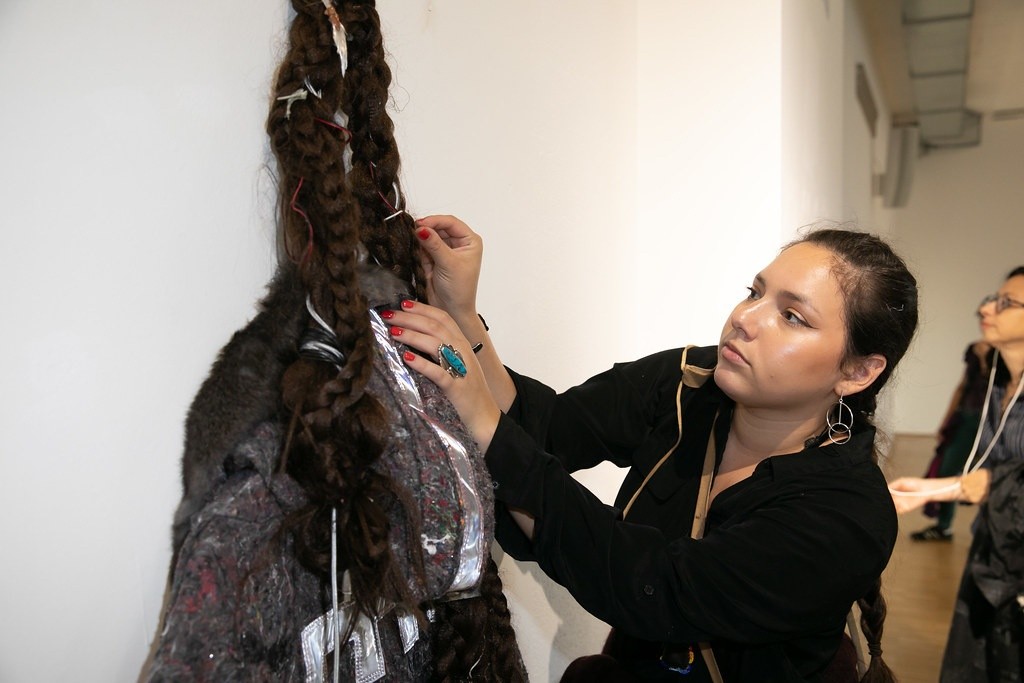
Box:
[911,524,953,542]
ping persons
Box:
[889,265,1024,683]
[379,214,921,683]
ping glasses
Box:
[982,292,1024,314]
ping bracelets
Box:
[473,313,491,353]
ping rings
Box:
[436,342,467,380]
